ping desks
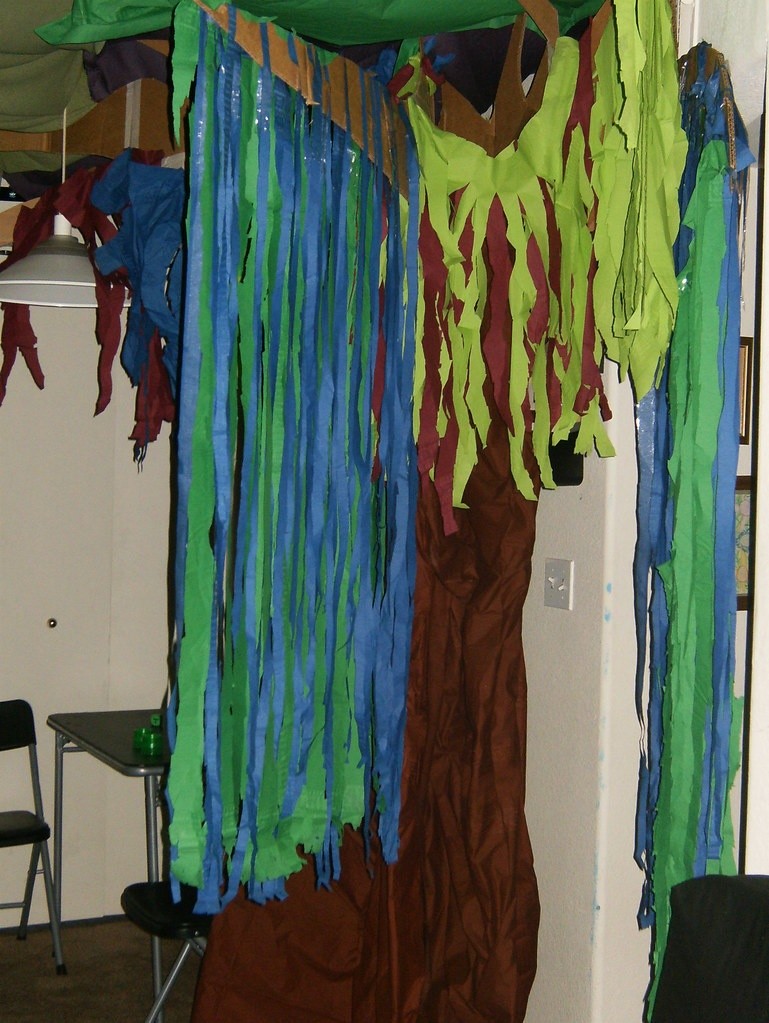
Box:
[46,708,205,1023]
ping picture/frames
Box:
[739,334,753,445]
[735,476,758,611]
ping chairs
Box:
[0,699,67,976]
[120,881,216,1023]
